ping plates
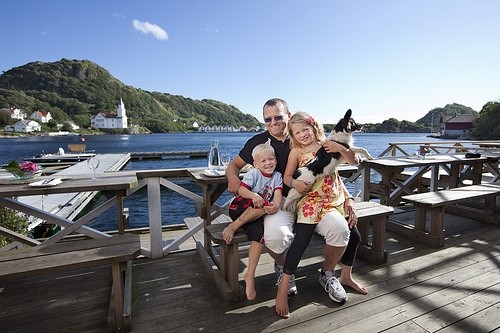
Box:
[240,164,255,173]
[204,170,226,177]
[28,180,62,187]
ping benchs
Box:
[205,202,395,300]
[0,235,142,333]
[401,183,500,245]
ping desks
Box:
[472,143,500,148]
[360,152,500,249]
[0,171,138,333]
[186,164,389,306]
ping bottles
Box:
[208,139,220,171]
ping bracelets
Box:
[290,179,294,188]
[346,196,354,200]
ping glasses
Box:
[264,112,287,122]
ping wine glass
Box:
[221,154,230,171]
[87,157,100,183]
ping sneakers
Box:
[318,267,348,303]
[273,260,298,296]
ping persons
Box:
[276,112,368,315]
[222,144,284,301]
[226,98,358,303]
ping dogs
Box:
[281,109,374,211]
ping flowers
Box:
[19,161,40,171]
